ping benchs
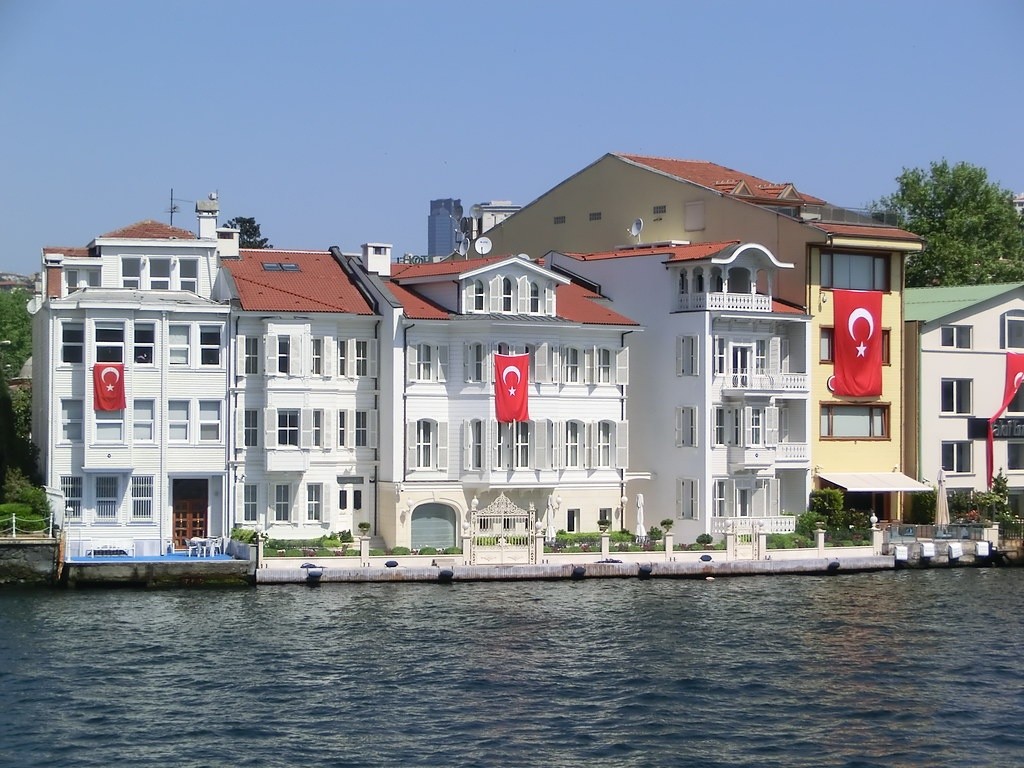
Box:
[91,534,136,558]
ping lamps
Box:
[255,521,264,539]
[402,498,413,514]
[462,522,470,536]
[870,513,879,530]
[536,518,543,535]
[724,518,732,532]
[471,495,478,514]
[758,519,766,533]
[618,496,628,509]
[554,496,562,509]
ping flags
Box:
[987,351,1024,489]
[831,287,883,396]
[494,351,530,422]
[94,363,126,411]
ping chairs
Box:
[186,536,222,558]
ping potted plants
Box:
[814,516,826,530]
[358,522,370,538]
[598,520,612,535]
[660,518,674,533]
